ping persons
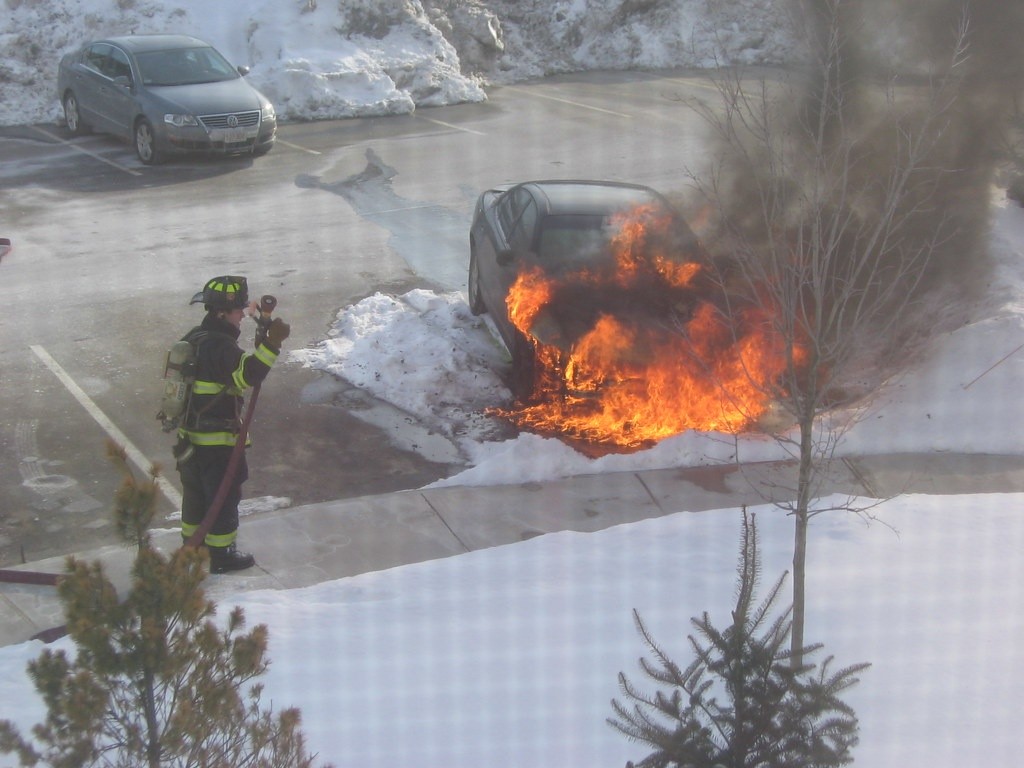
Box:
[162,275,290,574]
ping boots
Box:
[209,543,254,574]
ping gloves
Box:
[267,318,291,349]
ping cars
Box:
[57,34,282,166]
[467,178,720,396]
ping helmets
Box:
[202,275,250,310]
